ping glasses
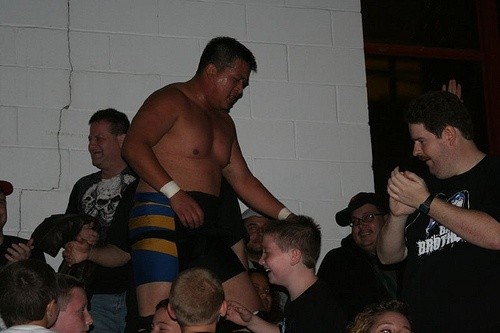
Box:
[348,213,384,227]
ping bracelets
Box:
[278,207,293,222]
[159,180,182,199]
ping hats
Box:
[335,191,389,227]
[0,179,13,196]
[241,209,262,219]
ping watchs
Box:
[418,193,436,215]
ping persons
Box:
[0,79,463,333]
[122,36,295,333]
[375,91,500,333]
